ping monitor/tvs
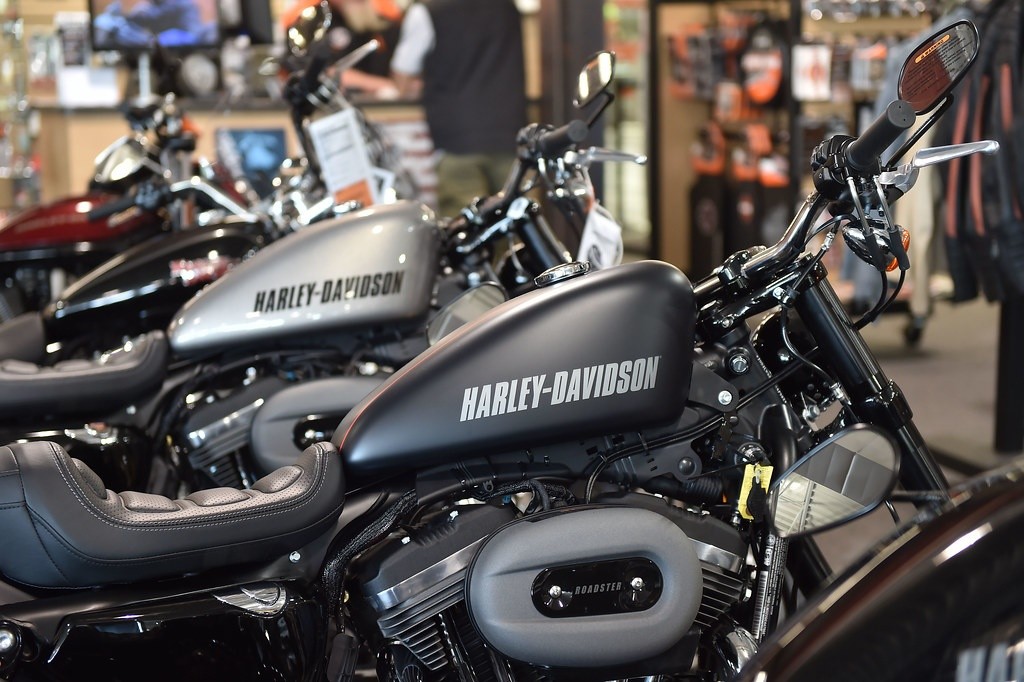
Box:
[87,0,222,52]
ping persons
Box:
[390,0,528,218]
[292,0,402,93]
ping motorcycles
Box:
[0,17,1024,682]
[0,35,214,317]
[0,0,418,364]
[0,49,650,496]
